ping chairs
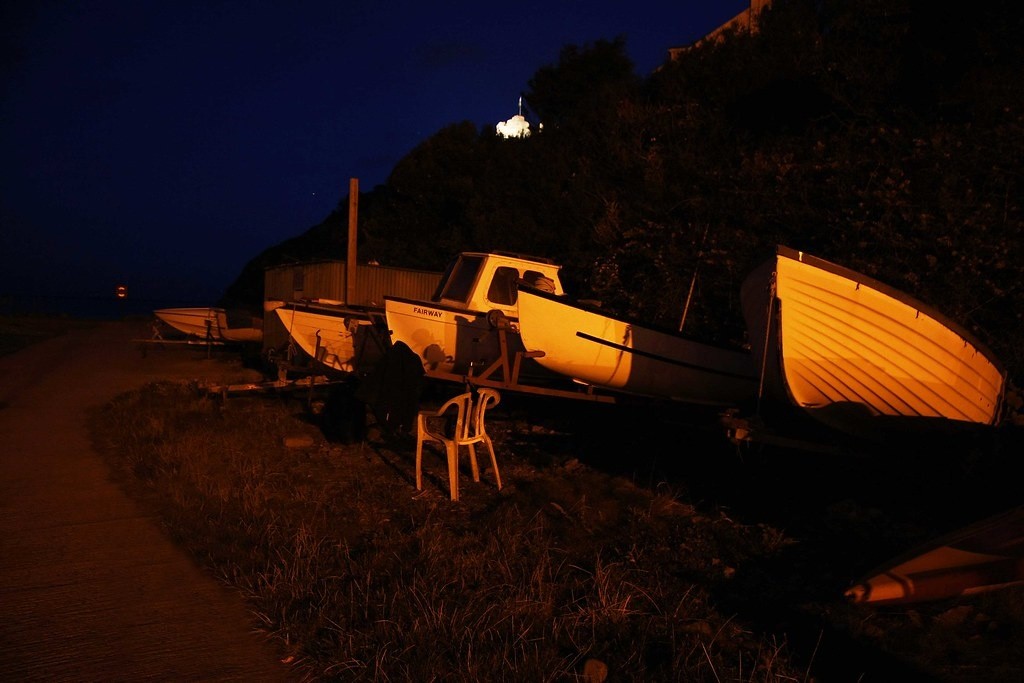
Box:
[415,388,502,503]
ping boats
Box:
[380,291,555,381]
[274,244,568,377]
[514,277,757,408]
[152,300,263,344]
[841,503,1024,609]
[740,244,1024,437]
